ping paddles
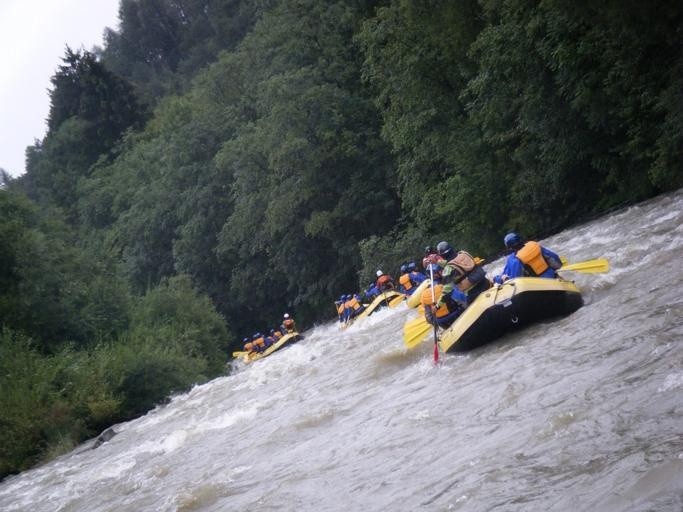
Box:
[405,316,427,333]
[406,324,432,348]
[555,259,609,272]
[233,352,250,357]
[429,263,443,364]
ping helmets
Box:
[341,293,358,300]
[424,241,452,255]
[426,264,443,273]
[243,328,275,342]
[400,263,416,272]
[504,234,520,246]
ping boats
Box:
[407,278,437,309]
[437,276,584,354]
[241,331,303,364]
[340,290,406,331]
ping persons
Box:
[332,262,395,323]
[241,312,297,357]
[397,240,491,330]
[492,230,563,284]
